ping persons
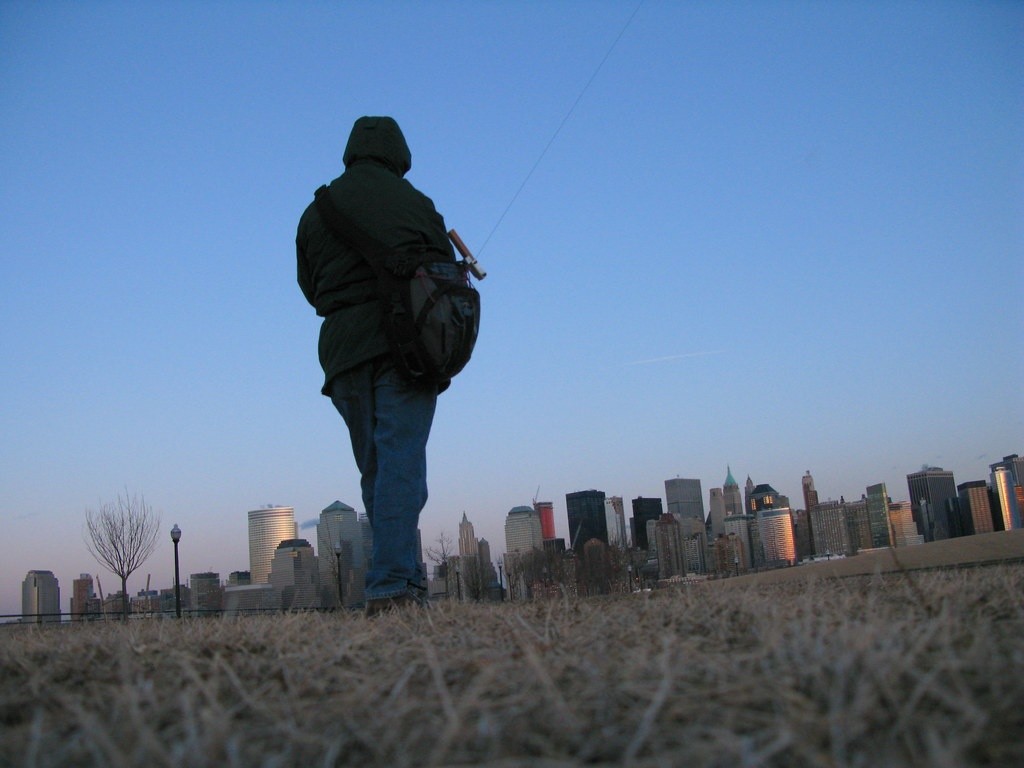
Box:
[295,115,455,620]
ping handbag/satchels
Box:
[367,243,479,393]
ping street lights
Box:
[496,557,506,602]
[825,548,831,563]
[506,571,514,601]
[454,565,462,604]
[331,542,345,611]
[733,556,740,577]
[628,564,633,588]
[170,523,185,619]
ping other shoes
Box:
[363,592,424,622]
